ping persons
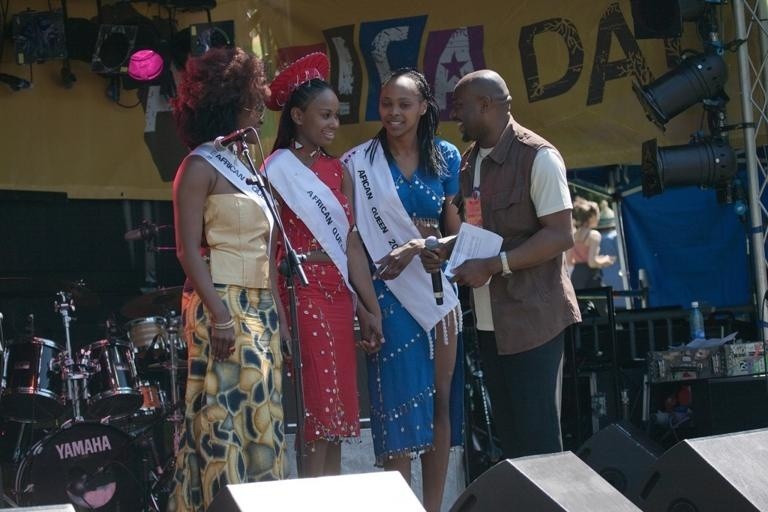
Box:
[572,196,612,289]
[260,52,386,478]
[339,68,461,512]
[169,48,292,511]
[419,70,583,458]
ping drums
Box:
[0,335,65,423]
[165,310,188,361]
[75,338,143,418]
[109,378,162,422]
[9,419,149,512]
[124,315,170,361]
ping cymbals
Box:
[138,357,187,372]
[121,286,183,318]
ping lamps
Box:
[629,0,738,206]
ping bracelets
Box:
[214,319,235,330]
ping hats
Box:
[598,200,616,229]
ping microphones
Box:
[212,126,253,151]
[423,236,452,307]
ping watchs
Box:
[497,250,514,278]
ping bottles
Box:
[669,404,690,438]
[689,302,705,339]
